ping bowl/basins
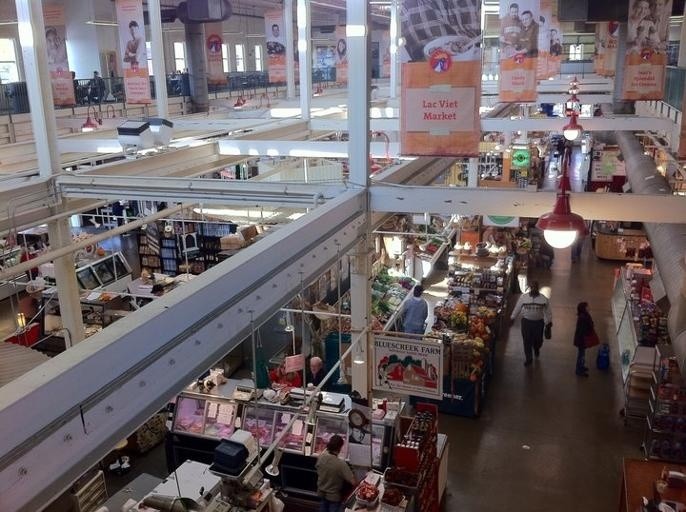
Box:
[423,35,474,61]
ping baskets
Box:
[356,483,381,508]
[379,489,406,512]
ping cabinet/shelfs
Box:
[589,129,686,512]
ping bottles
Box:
[208,382,212,389]
[198,380,203,390]
[137,235,176,275]
[378,398,388,414]
[308,383,313,390]
[17,312,26,327]
[661,466,668,480]
[396,411,434,449]
[174,223,194,233]
[97,247,104,255]
[179,261,194,273]
[194,224,228,273]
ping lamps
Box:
[535,176,584,250]
[142,116,174,149]
[81,105,102,133]
[563,112,583,141]
[117,118,155,158]
[566,77,580,117]
[233,87,246,109]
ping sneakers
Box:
[523,358,533,365]
[533,347,540,357]
[576,366,589,371]
[576,370,589,378]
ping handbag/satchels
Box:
[545,322,552,340]
[583,334,600,347]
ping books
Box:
[136,224,254,283]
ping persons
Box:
[44,26,67,65]
[574,301,600,373]
[397,3,484,64]
[554,137,565,164]
[401,285,429,342]
[206,34,222,62]
[307,355,335,394]
[550,27,560,54]
[497,1,540,60]
[315,435,357,511]
[267,24,287,52]
[70,72,84,100]
[336,39,348,64]
[624,0,674,55]
[122,22,145,67]
[270,358,301,388]
[508,280,554,367]
[84,69,109,102]
[564,141,574,165]
